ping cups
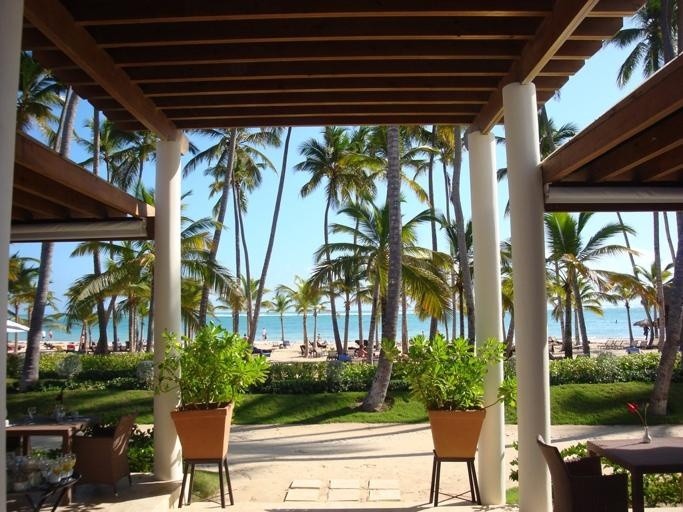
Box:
[4,420,9,427]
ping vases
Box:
[642,427,651,443]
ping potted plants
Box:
[378,333,516,459]
[152,317,272,459]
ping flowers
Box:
[626,400,650,427]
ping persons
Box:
[261,327,266,339]
[242,333,247,339]
[358,345,367,357]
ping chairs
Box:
[71,411,136,496]
[535,434,628,511]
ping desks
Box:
[585,435,682,511]
[5,419,88,511]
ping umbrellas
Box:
[6,320,29,332]
